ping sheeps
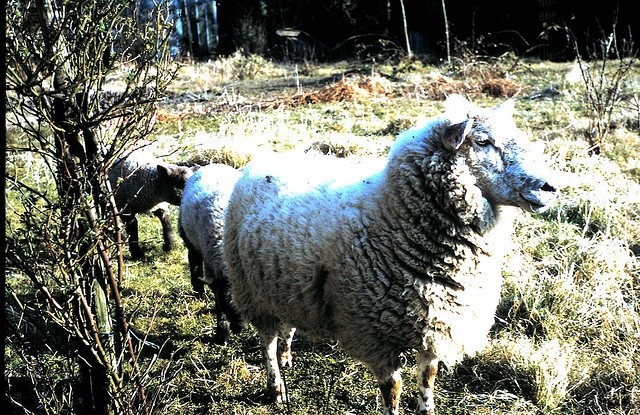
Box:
[222,88,561,414]
[58,142,194,263]
[178,164,245,338]
